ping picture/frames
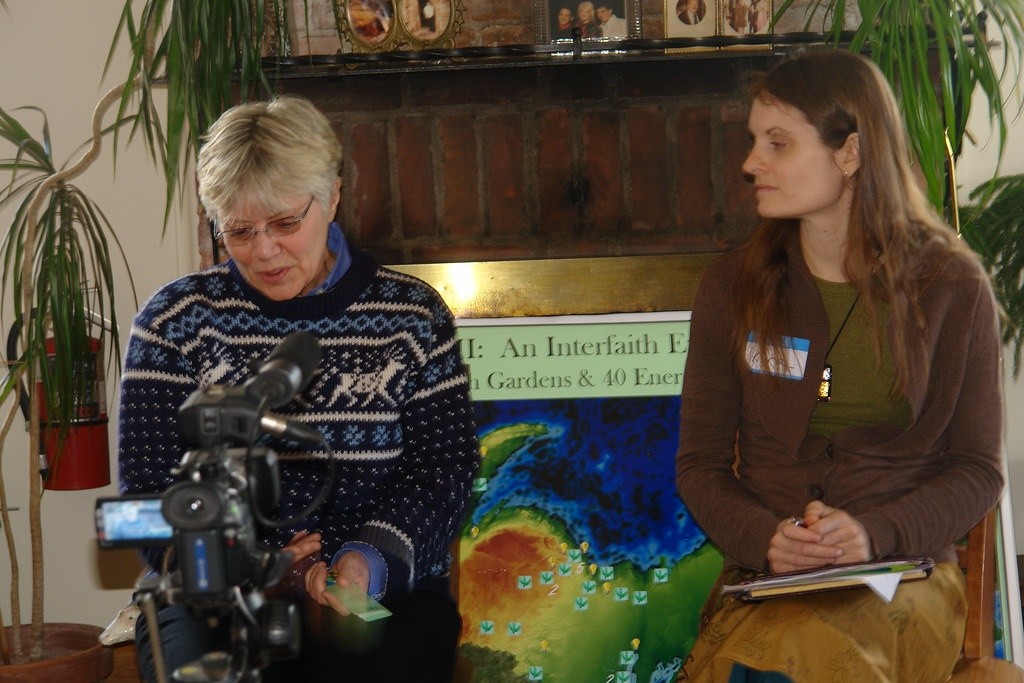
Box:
[194,0,291,65]
[533,0,643,46]
[332,0,466,54]
[663,0,773,54]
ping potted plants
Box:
[0,0,298,683]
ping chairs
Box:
[943,490,1024,683]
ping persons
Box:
[116,95,483,682]
[670,45,1006,683]
[678,0,702,25]
[348,0,387,40]
[731,0,769,33]
[112,503,152,538]
[553,0,627,39]
[423,1,435,33]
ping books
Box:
[719,556,934,605]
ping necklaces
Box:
[815,247,888,402]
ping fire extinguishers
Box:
[4,279,123,492]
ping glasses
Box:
[213,196,316,246]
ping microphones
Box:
[255,333,322,409]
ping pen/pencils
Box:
[791,514,809,530]
